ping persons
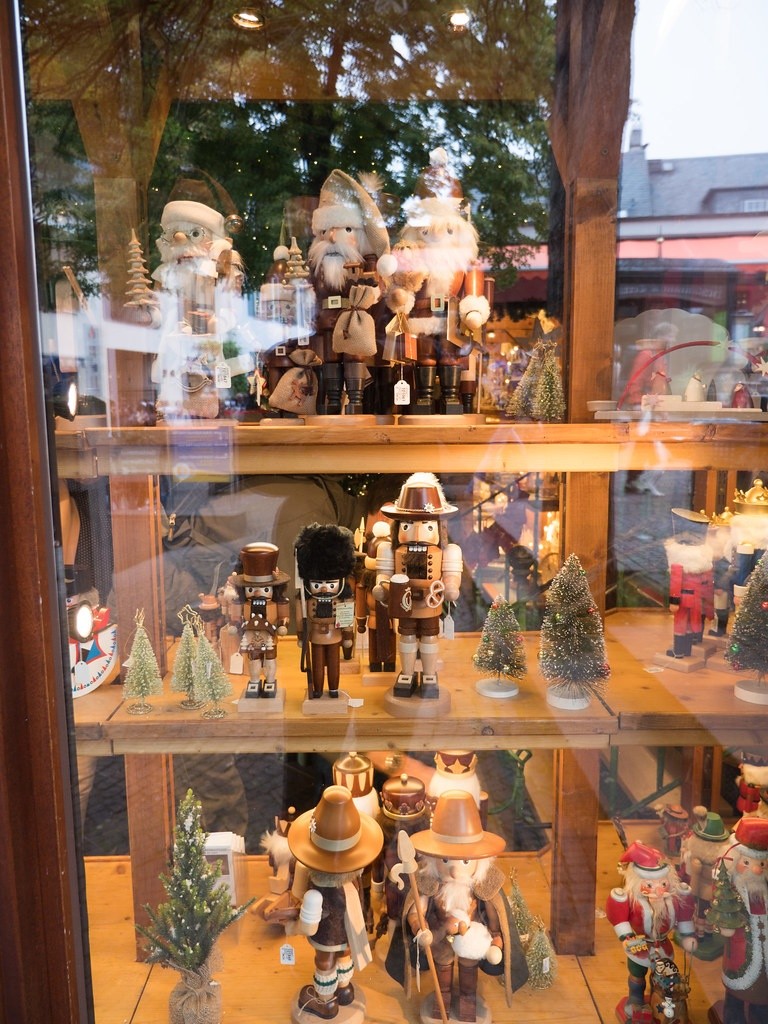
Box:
[616,321,679,497]
[258,751,526,1024]
[185,473,464,710]
[665,477,768,681]
[149,143,496,421]
[81,473,552,855]
[605,744,768,1024]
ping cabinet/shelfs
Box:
[47,407,768,1024]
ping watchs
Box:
[383,752,405,777]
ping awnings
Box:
[471,235,768,286]
